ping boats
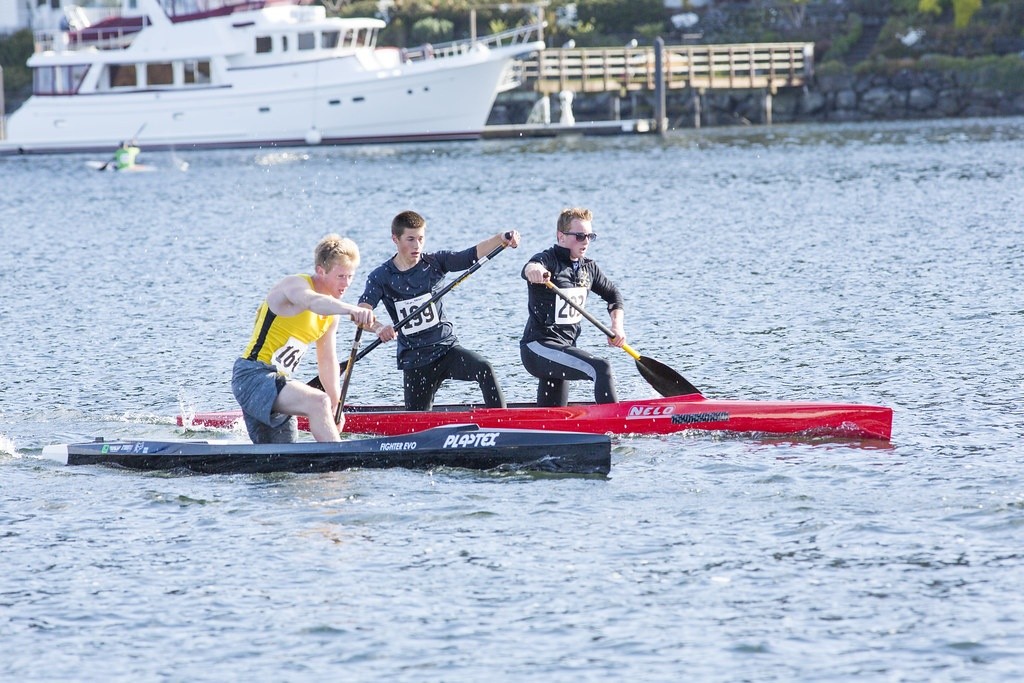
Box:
[7,0,554,160]
[184,391,898,440]
[57,422,615,474]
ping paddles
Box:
[303,232,518,393]
[530,271,704,398]
[333,315,377,426]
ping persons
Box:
[519,208,626,408]
[232,234,373,443]
[355,211,522,407]
[112,140,141,172]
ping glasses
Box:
[563,233,596,241]
